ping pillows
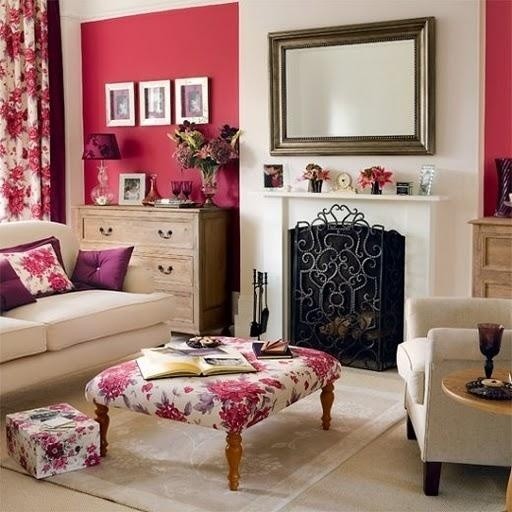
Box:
[71,245,136,292]
[1,255,39,317]
[0,235,67,277]
[0,242,77,298]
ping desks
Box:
[440,364,512,510]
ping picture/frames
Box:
[103,81,137,128]
[116,172,149,206]
[138,78,172,127]
[173,75,210,126]
[416,164,436,196]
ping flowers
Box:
[301,162,331,182]
[357,165,397,188]
[169,118,243,165]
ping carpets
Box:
[2,368,408,511]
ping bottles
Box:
[140,173,162,207]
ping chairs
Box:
[396,294,511,499]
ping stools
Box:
[5,401,102,483]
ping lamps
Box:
[82,131,123,207]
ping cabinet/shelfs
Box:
[468,215,512,298]
[72,205,236,335]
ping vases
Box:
[370,181,384,195]
[309,179,325,192]
[196,163,222,208]
[493,154,511,216]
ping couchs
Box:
[1,220,177,410]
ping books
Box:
[252,339,293,359]
[135,345,257,381]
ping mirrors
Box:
[267,14,437,155]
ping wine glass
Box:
[170,179,192,200]
[476,321,504,382]
